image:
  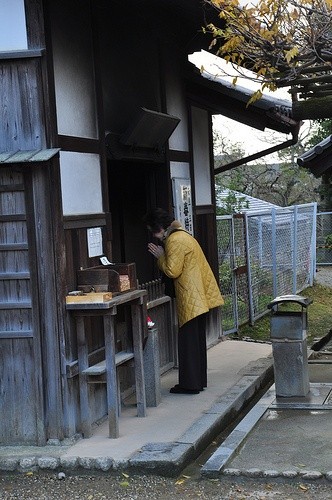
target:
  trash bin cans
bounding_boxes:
[267,292,314,398]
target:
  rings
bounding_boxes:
[153,250,154,253]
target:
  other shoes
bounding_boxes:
[170,384,203,394]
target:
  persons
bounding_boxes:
[148,208,224,394]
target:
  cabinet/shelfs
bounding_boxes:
[63,287,147,439]
